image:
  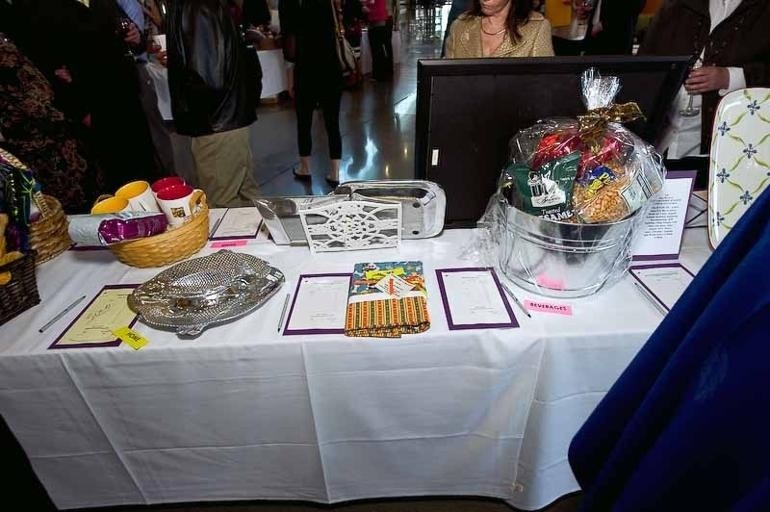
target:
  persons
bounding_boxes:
[0,1,769,212]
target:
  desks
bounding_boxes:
[551,24,587,56]
[144,48,288,122]
[0,207,714,511]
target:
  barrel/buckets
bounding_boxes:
[493,202,650,299]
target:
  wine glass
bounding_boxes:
[108,13,136,56]
[679,64,701,117]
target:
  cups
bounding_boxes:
[90,176,207,230]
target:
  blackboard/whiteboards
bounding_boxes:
[416,55,696,229]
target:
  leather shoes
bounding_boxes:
[292,167,311,182]
[325,175,339,187]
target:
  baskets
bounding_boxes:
[93,191,209,267]
[0,149,73,325]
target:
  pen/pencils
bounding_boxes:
[278,293,290,333]
[499,282,532,319]
[38,295,86,333]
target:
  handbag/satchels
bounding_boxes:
[335,34,361,86]
